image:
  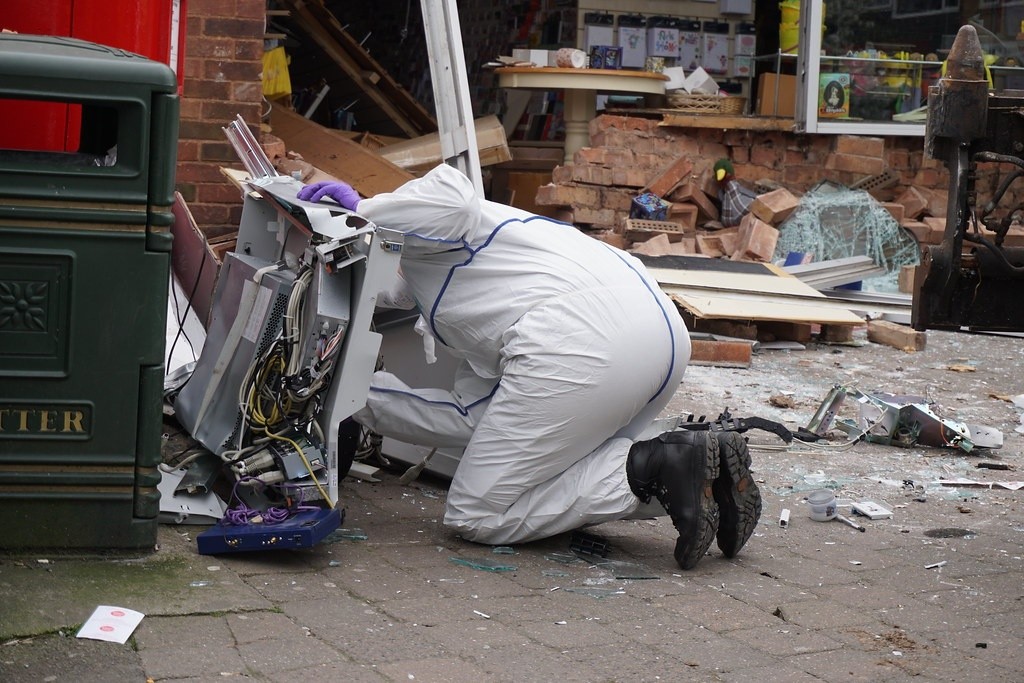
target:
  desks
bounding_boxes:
[745,51,1024,118]
[493,66,671,221]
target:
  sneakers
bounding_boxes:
[626,429,722,571]
[709,432,762,558]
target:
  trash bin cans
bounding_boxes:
[0,32,182,558]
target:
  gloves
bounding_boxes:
[296,181,361,213]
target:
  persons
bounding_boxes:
[294,163,762,569]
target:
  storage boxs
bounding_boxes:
[818,72,851,119]
[378,113,513,177]
[628,193,674,221]
[584,24,757,79]
[663,66,687,90]
[755,71,796,118]
[683,64,721,94]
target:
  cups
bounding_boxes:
[651,57,664,73]
[645,57,653,72]
[557,48,586,68]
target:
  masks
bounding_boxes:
[387,274,417,311]
